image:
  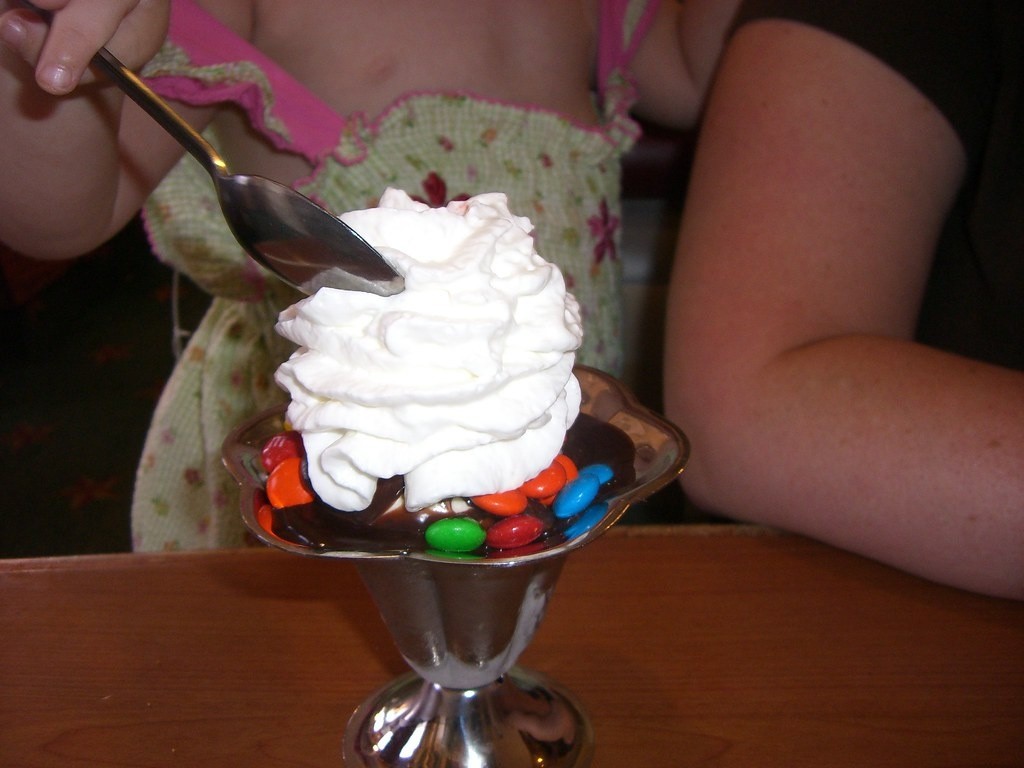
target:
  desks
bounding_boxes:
[0,524,1024,768]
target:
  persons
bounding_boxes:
[664,0,1024,603]
[0,0,743,553]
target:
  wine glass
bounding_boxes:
[217,375,690,768]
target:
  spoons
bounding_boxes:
[23,2,405,297]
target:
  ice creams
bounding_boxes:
[252,185,641,552]
